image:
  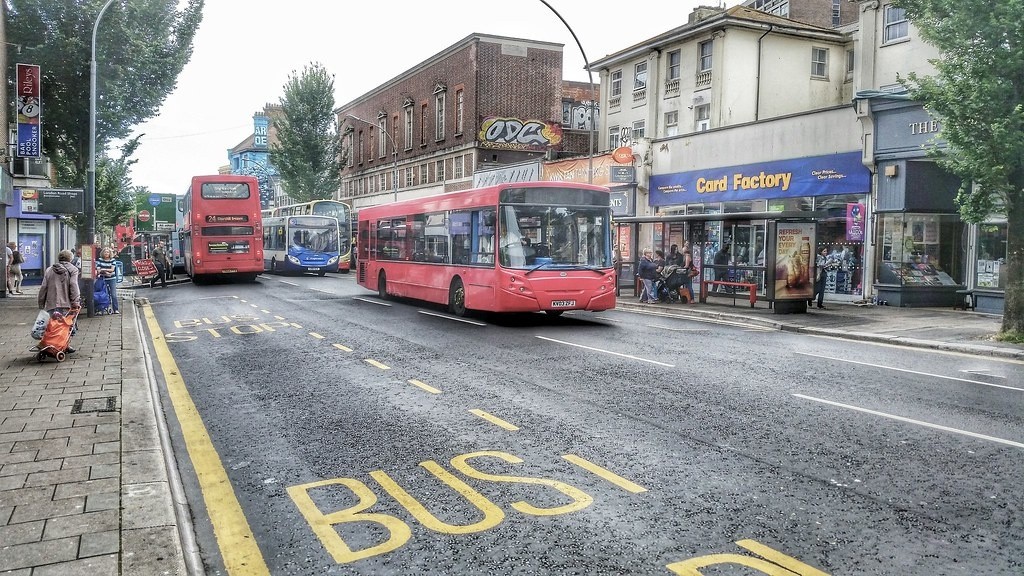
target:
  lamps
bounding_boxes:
[599,68,609,76]
[717,28,725,38]
[650,50,661,58]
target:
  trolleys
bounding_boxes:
[29,305,82,362]
[93,274,113,316]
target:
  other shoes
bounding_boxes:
[16,290,24,294]
[64,346,76,353]
[115,310,120,314]
[95,311,103,316]
[9,290,14,295]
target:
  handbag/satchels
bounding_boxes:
[31,310,50,339]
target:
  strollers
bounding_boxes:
[657,267,690,305]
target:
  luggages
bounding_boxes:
[30,306,81,362]
[94,274,113,315]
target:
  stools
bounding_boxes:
[953,290,975,311]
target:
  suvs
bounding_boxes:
[114,245,148,276]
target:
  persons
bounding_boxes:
[71,247,120,314]
[639,244,695,304]
[491,223,528,264]
[712,244,733,294]
[6,242,23,298]
[808,246,833,310]
[463,234,489,263]
[37,250,80,354]
[580,244,623,295]
[151,240,171,288]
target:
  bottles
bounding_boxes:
[800,236,811,283]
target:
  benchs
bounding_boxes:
[702,280,756,307]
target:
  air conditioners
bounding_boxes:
[0,148,11,164]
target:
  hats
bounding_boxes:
[7,242,16,247]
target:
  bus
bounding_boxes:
[172,173,265,286]
[134,231,172,253]
[262,215,341,276]
[356,180,617,319]
[261,199,352,275]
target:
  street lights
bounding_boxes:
[346,112,398,202]
[241,158,277,208]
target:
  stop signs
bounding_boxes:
[138,210,150,222]
